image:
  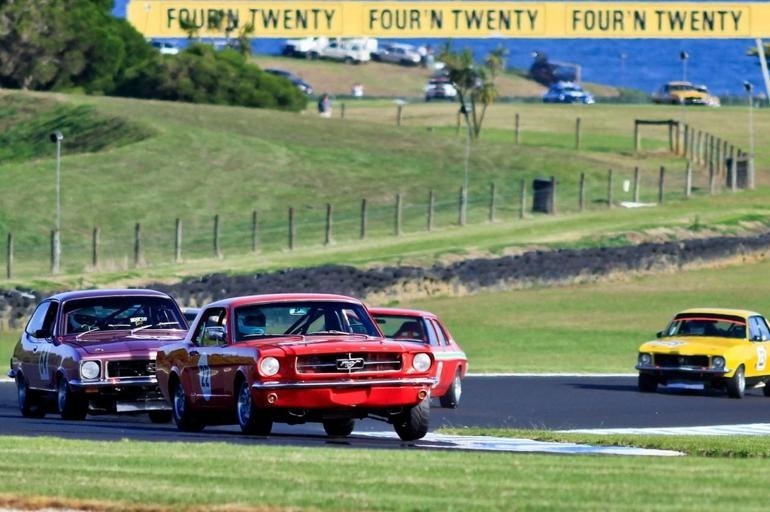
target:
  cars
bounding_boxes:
[635,307,770,398]
[424,77,457,101]
[652,81,708,105]
[7,288,191,423]
[320,308,470,409]
[696,86,722,107]
[265,70,314,98]
[541,83,594,104]
[370,43,422,67]
[177,307,264,338]
[154,293,438,441]
[530,64,582,81]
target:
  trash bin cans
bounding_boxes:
[726,158,747,189]
[533,178,552,213]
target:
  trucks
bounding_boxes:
[285,38,378,61]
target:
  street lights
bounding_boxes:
[619,53,627,98]
[744,80,754,188]
[680,51,689,81]
[49,129,64,275]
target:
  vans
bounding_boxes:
[152,42,179,55]
[319,42,370,65]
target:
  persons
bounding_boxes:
[317,92,335,120]
